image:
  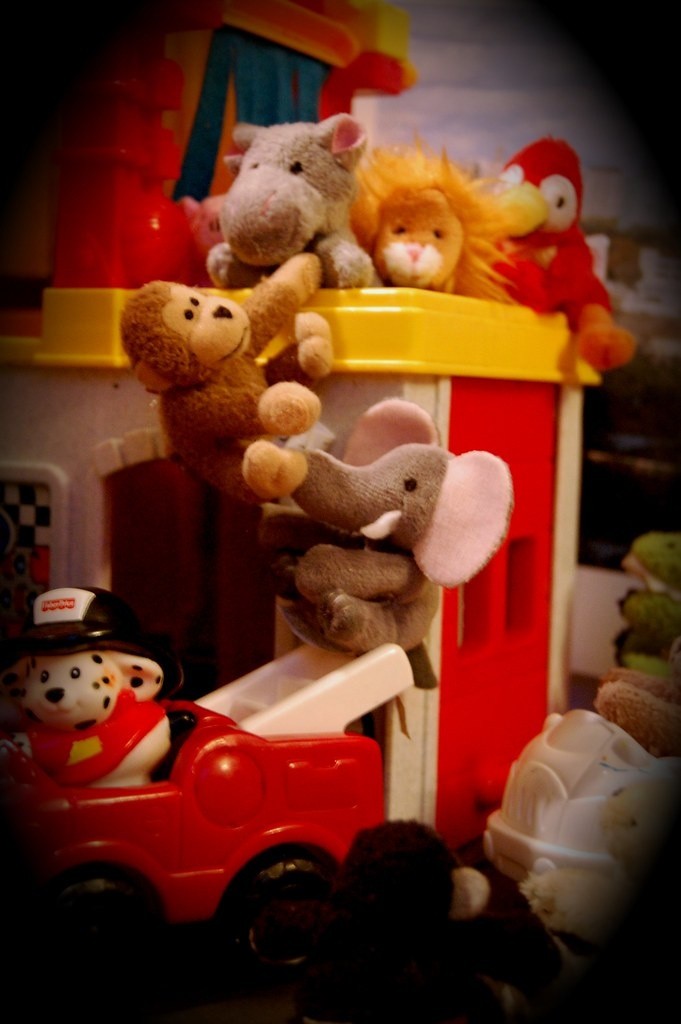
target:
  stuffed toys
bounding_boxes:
[518,757,681,946]
[207,112,382,287]
[353,145,511,302]
[0,587,186,784]
[183,194,225,247]
[249,399,513,687]
[296,821,490,1024]
[118,254,333,504]
[597,531,680,754]
[497,136,635,370]
